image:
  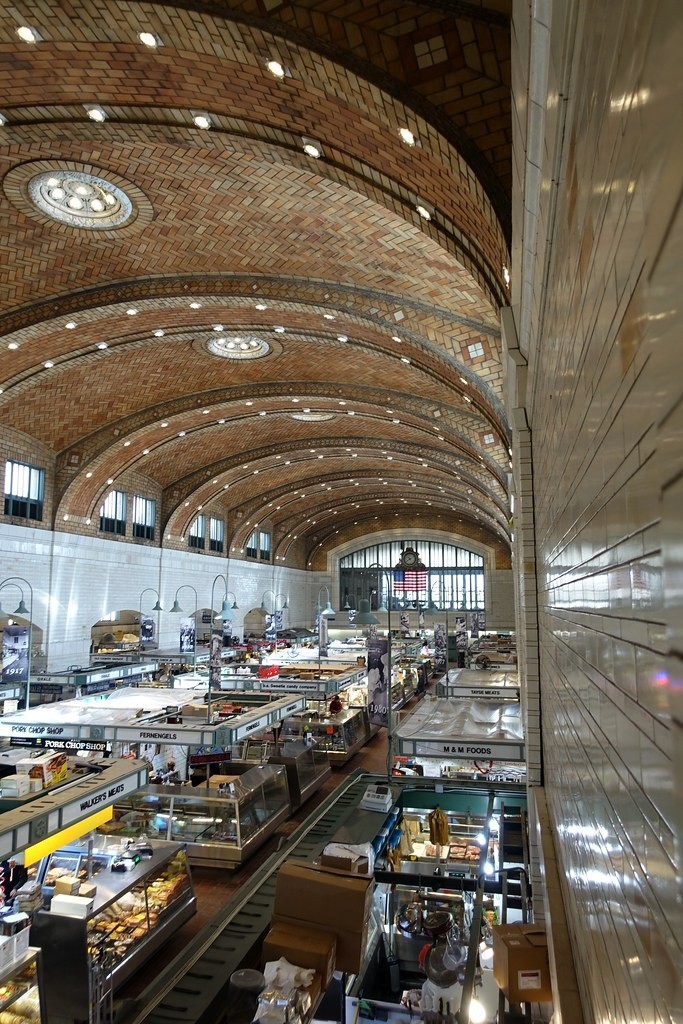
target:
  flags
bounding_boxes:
[393,570,426,592]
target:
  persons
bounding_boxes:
[0,859,27,910]
[422,638,429,652]
[285,643,292,648]
[391,665,393,676]
[152,666,206,682]
[329,695,343,715]
[417,670,424,702]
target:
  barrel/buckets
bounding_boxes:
[227,969,265,1024]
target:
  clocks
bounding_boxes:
[402,551,417,566]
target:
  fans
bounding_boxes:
[473,654,491,671]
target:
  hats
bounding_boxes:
[335,695,339,700]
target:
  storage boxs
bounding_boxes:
[50,875,98,916]
[181,704,212,716]
[496,638,510,644]
[256,840,374,1024]
[299,672,314,680]
[492,923,553,1003]
[0,748,67,798]
[119,826,141,837]
